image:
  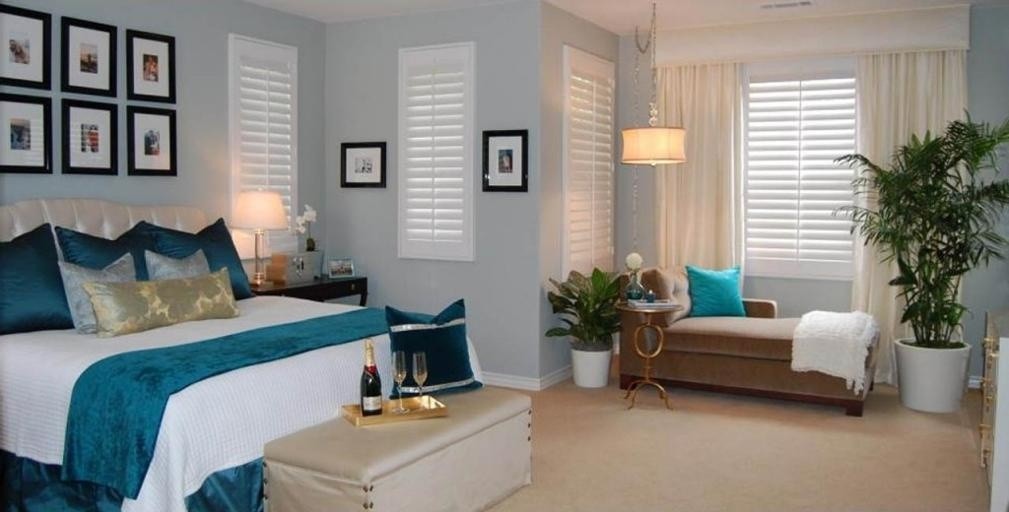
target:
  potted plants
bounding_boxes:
[826,101,1009,416]
[543,265,629,389]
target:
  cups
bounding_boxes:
[646,294,656,302]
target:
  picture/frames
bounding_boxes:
[339,139,389,190]
[480,127,531,195]
[60,98,121,178]
[324,254,359,281]
[58,4,122,97]
[126,103,178,178]
[0,91,58,177]
[126,7,185,106]
[1,1,54,92]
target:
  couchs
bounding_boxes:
[621,263,885,418]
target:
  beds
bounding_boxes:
[2,193,494,512]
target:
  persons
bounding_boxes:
[7,35,162,156]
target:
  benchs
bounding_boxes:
[260,384,538,511]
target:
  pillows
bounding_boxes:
[639,263,694,327]
[0,221,263,337]
[381,295,487,400]
[684,264,750,317]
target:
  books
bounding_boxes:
[627,298,680,311]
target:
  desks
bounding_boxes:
[614,297,685,414]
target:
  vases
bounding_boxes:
[302,251,324,279]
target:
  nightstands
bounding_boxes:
[250,277,374,308]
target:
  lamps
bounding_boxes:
[619,3,697,169]
[227,184,289,286]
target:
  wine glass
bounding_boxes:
[409,352,433,411]
[389,351,412,414]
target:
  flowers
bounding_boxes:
[293,201,323,253]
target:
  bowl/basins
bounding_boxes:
[627,290,643,299]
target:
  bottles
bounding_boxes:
[359,338,385,418]
